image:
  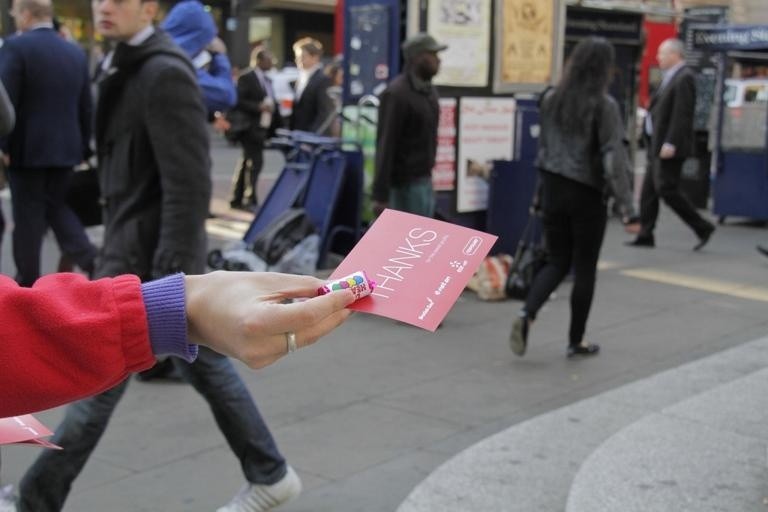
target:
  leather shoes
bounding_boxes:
[626,237,656,248]
[694,223,715,251]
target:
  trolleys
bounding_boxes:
[211,94,381,281]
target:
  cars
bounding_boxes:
[226,64,299,135]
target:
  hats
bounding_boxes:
[404,35,448,55]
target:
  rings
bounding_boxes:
[287,332,298,355]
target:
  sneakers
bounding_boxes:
[568,342,600,357]
[217,465,302,512]
[510,312,528,355]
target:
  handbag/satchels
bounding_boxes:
[69,165,103,228]
[505,240,552,302]
[225,107,251,142]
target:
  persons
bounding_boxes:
[160,1,236,109]
[1,0,101,287]
[370,31,448,217]
[0,0,302,512]
[224,47,284,212]
[209,110,229,133]
[623,38,716,251]
[509,35,641,360]
[0,270,356,418]
[287,38,345,134]
